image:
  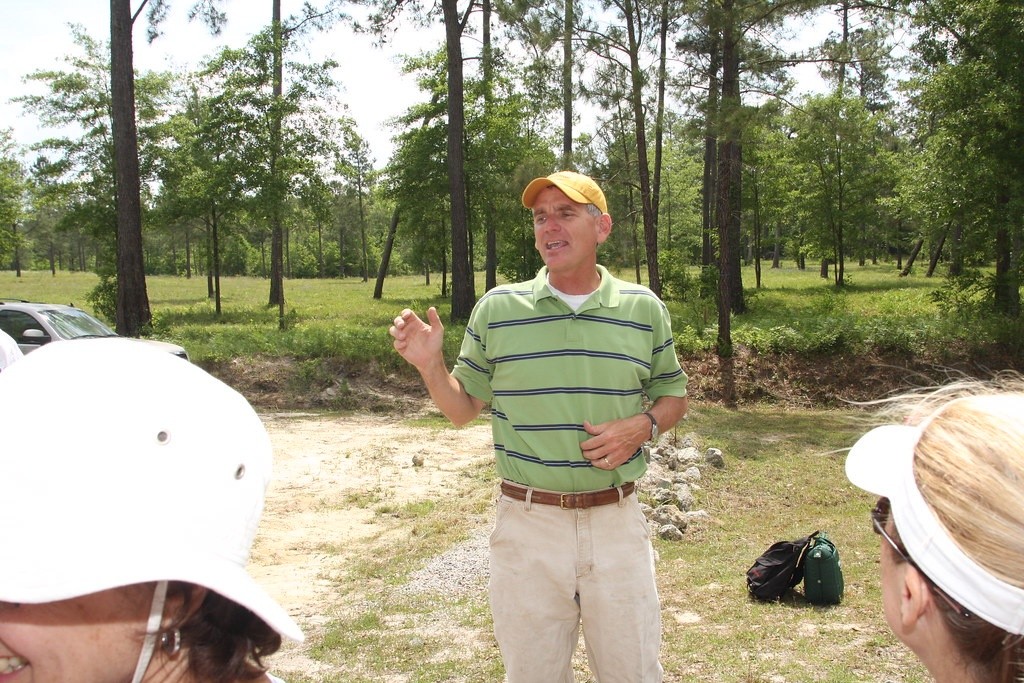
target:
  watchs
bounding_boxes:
[645,411,658,442]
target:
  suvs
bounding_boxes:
[0,297,190,363]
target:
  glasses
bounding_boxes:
[872,494,967,621]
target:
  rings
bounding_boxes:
[604,458,612,468]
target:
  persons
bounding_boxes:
[845,372,1024,683]
[0,336,304,683]
[388,172,690,683]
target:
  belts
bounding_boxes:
[497,482,638,509]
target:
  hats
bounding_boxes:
[844,402,1024,632]
[522,170,606,213]
[0,340,303,638]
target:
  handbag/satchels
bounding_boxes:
[747,529,844,607]
